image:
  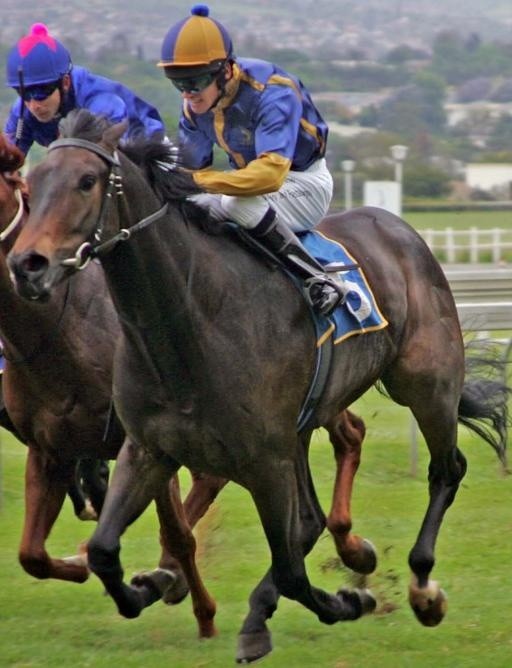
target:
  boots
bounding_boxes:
[253,211,349,320]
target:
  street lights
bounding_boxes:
[340,158,356,212]
[388,144,409,220]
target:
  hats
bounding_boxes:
[6,22,74,87]
[156,3,233,79]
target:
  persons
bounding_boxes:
[156,4,347,317]
[3,22,166,165]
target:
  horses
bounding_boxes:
[8,111,512,663]
[1,135,380,642]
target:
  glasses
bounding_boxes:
[167,39,232,95]
[14,81,60,105]
